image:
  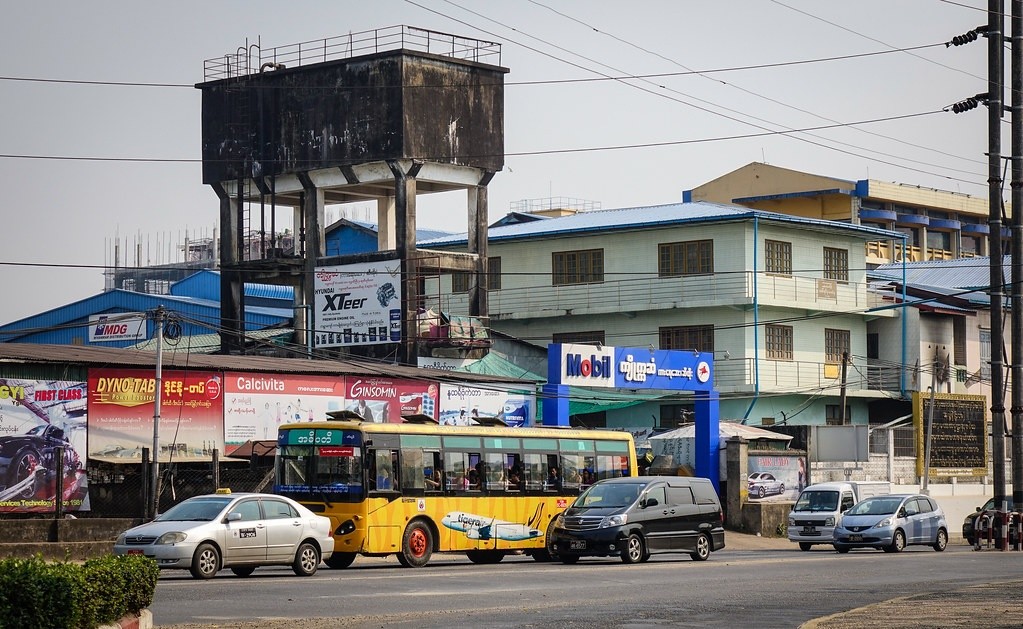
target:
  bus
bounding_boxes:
[251,410,639,569]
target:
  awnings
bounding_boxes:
[450,352,673,420]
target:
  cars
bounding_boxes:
[963,496,1013,545]
[833,494,949,553]
[748,471,786,498]
[114,488,335,579]
[0,425,80,502]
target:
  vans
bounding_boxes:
[555,476,725,564]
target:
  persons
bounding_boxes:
[444,408,478,426]
[377,463,623,491]
[798,460,806,496]
[263,398,313,439]
[354,399,374,422]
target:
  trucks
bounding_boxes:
[787,481,891,551]
